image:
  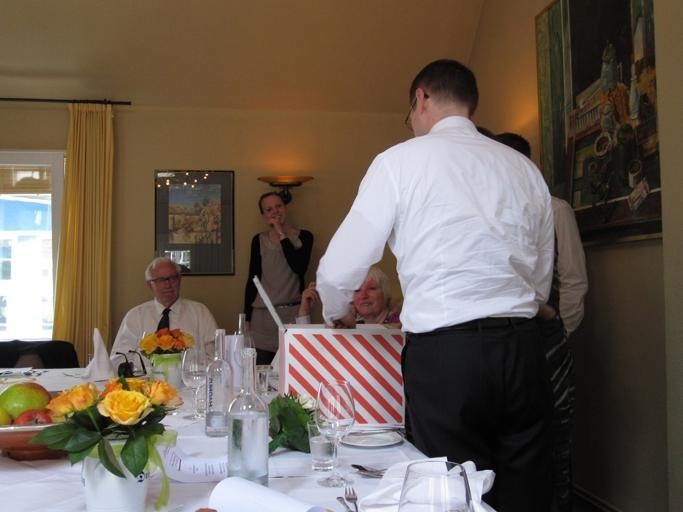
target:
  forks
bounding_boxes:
[344,487,358,511]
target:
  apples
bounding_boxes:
[0,410,12,425]
[1,381,52,422]
[14,409,51,427]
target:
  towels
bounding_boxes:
[84,328,115,382]
[360,457,496,507]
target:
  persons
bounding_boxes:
[296,267,391,325]
[496,129,590,511]
[316,58,553,512]
[244,192,315,366]
[110,257,220,379]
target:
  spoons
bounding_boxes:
[350,464,385,476]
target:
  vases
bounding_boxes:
[82,439,170,512]
[150,353,182,386]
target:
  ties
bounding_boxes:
[157,308,171,332]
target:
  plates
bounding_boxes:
[339,431,402,447]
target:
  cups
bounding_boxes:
[255,365,268,393]
[306,420,337,471]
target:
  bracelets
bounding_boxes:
[277,232,285,237]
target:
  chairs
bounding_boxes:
[0,340,81,368]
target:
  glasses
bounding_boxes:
[405,93,429,135]
[148,275,180,283]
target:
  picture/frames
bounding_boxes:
[155,168,236,276]
[536,4,670,243]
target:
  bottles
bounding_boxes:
[205,328,232,441]
[236,312,251,352]
[224,348,270,489]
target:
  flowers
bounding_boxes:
[28,372,178,479]
[136,327,194,356]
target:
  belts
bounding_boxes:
[406,317,529,335]
[275,301,301,308]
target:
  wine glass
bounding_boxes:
[181,350,205,420]
[397,461,475,511]
[233,329,255,389]
[315,381,354,487]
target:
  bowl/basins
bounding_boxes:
[0,423,66,461]
